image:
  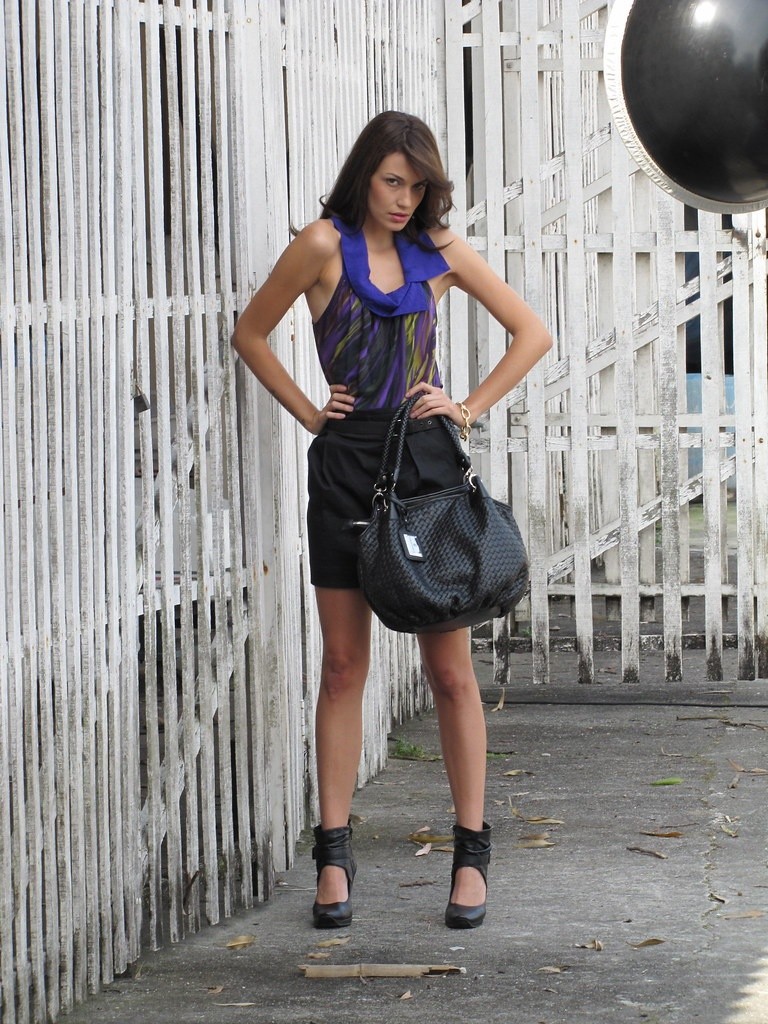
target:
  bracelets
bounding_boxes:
[454,401,472,441]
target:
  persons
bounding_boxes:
[230,111,554,928]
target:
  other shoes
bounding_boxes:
[313,823,357,929]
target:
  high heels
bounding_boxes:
[446,823,492,931]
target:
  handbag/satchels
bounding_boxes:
[361,391,529,633]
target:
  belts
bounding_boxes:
[329,417,440,436]
